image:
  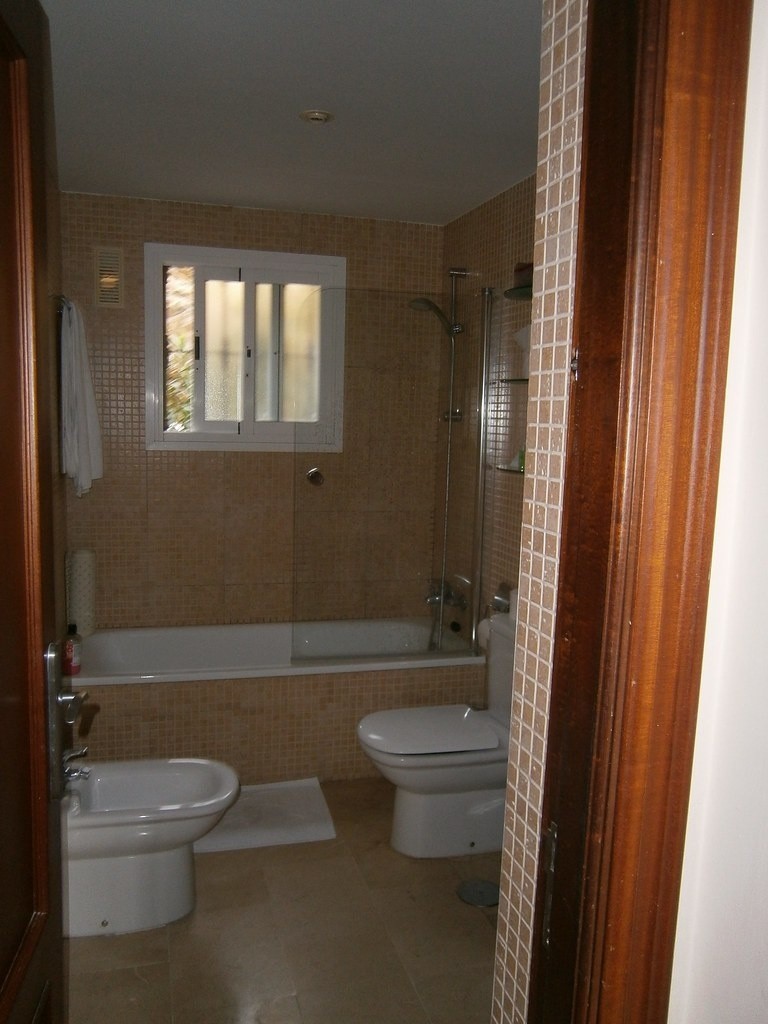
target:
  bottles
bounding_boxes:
[60,623,83,676]
[518,440,526,473]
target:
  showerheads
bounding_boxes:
[407,295,457,340]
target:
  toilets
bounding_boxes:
[355,618,518,860]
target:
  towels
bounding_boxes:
[56,300,105,500]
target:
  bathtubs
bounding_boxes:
[56,616,489,687]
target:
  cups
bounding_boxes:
[515,263,533,286]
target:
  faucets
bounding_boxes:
[58,742,92,789]
[424,578,457,609]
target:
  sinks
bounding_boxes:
[67,758,242,856]
